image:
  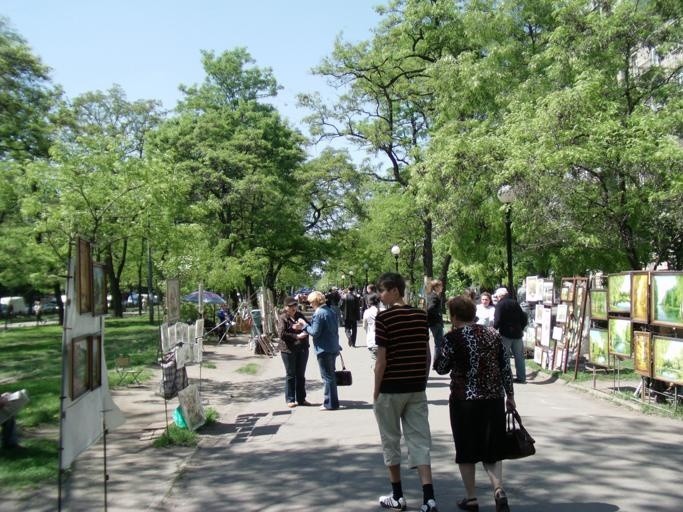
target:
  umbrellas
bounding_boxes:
[179,289,225,305]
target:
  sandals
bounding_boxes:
[457,498,479,512]
[494,487,510,512]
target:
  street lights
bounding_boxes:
[320,264,374,295]
[494,182,520,307]
[389,244,401,277]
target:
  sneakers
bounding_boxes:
[419,498,437,512]
[379,496,406,511]
[298,400,311,405]
[287,402,296,407]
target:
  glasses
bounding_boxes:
[378,288,387,294]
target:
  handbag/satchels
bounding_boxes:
[334,352,352,385]
[503,407,535,459]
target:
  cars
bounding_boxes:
[0,283,160,325]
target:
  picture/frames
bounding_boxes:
[608,316,633,359]
[650,271,683,329]
[89,331,101,391]
[589,328,609,367]
[630,271,649,324]
[72,334,89,402]
[78,237,91,315]
[608,273,630,313]
[91,262,108,316]
[653,335,683,386]
[633,331,652,377]
[525,276,583,370]
[589,289,608,320]
[167,279,180,321]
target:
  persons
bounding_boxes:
[291,291,342,411]
[215,304,226,343]
[372,272,441,512]
[31,300,45,327]
[430,296,517,511]
[275,295,312,409]
[319,277,527,385]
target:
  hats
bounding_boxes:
[283,295,298,307]
